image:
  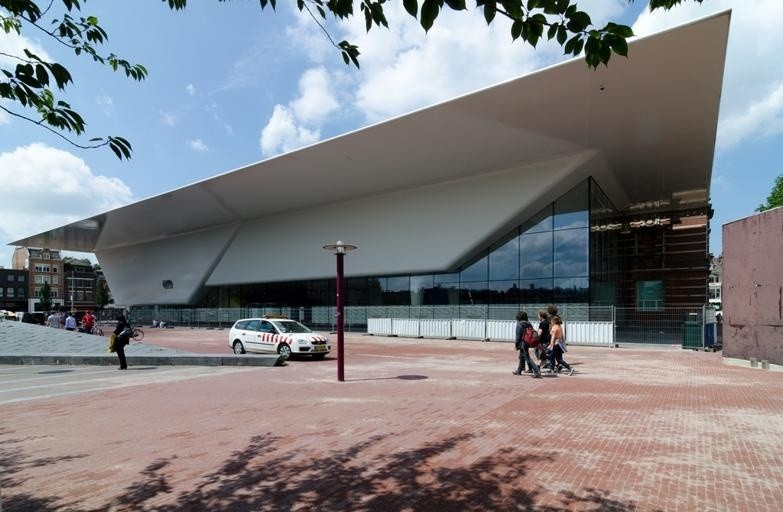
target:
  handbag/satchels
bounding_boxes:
[117,327,132,343]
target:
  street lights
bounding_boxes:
[321,240,358,381]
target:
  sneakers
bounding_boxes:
[512,362,574,378]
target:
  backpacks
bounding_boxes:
[522,328,538,347]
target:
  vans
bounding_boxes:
[20,313,45,325]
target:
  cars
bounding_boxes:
[228,315,332,360]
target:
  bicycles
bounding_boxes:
[130,327,144,341]
[90,327,103,336]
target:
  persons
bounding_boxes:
[59,312,64,328]
[81,310,94,334]
[538,306,574,376]
[46,310,58,328]
[113,315,129,370]
[64,312,76,331]
[511,311,541,378]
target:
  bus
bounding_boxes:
[0,310,17,321]
